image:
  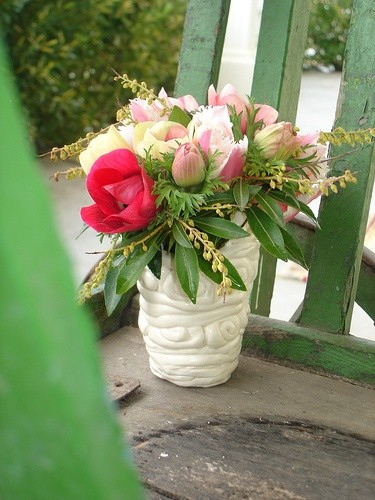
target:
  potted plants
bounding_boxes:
[30,65,375,318]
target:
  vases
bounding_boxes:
[134,212,263,388]
